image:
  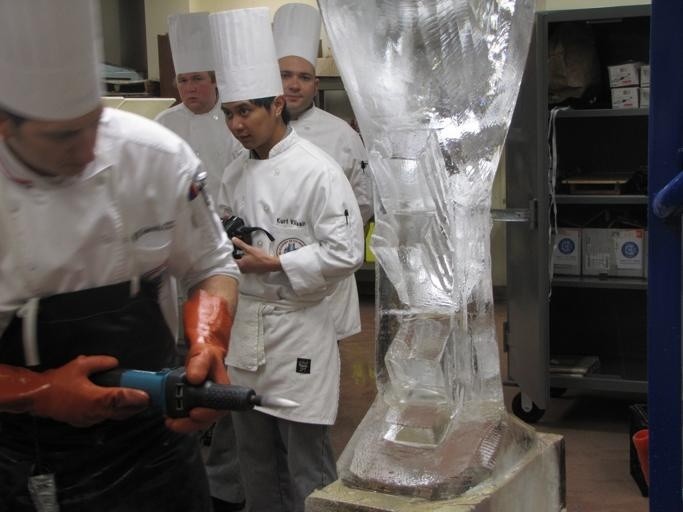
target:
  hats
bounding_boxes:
[165,2,321,103]
[0,1,103,123]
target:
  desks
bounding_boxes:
[294,425,567,512]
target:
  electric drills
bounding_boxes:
[87,367,301,420]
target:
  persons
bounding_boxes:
[153,11,252,204]
[0,1,241,512]
[206,6,364,512]
[271,3,370,339]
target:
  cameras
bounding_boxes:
[222,216,252,259]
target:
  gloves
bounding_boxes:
[0,356,151,427]
[166,290,232,438]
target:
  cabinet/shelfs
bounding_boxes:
[527,2,653,396]
[313,74,381,282]
